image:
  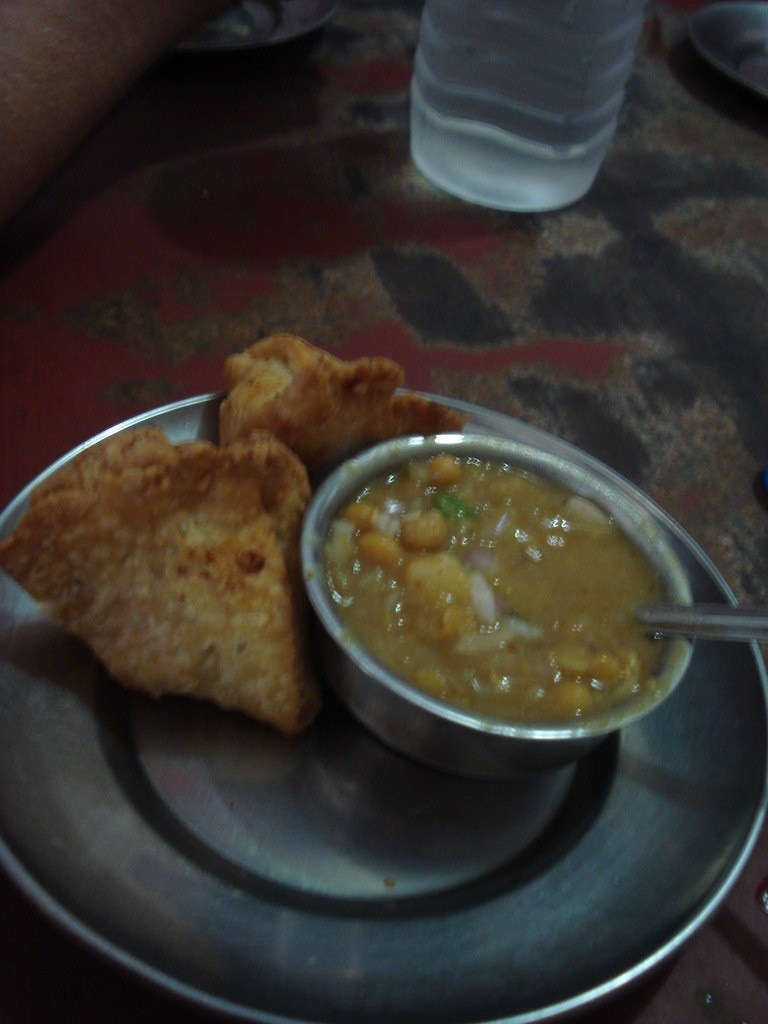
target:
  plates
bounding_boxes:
[691,1,768,103]
[3,379,768,1024]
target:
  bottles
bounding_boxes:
[404,1,650,215]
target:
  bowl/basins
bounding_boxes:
[298,435,704,784]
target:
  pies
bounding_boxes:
[215,334,467,492]
[0,426,327,730]
